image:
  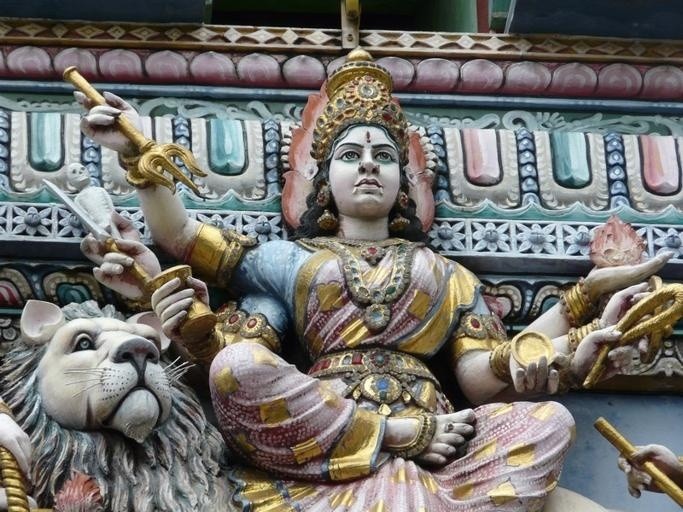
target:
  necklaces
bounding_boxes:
[301,237,424,333]
[331,234,409,262]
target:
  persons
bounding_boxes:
[71,45,673,511]
[0,394,40,511]
[617,440,682,500]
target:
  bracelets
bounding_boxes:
[557,276,596,328]
[182,331,228,364]
[134,297,153,313]
[118,146,164,189]
[489,341,519,385]
[567,318,600,350]
